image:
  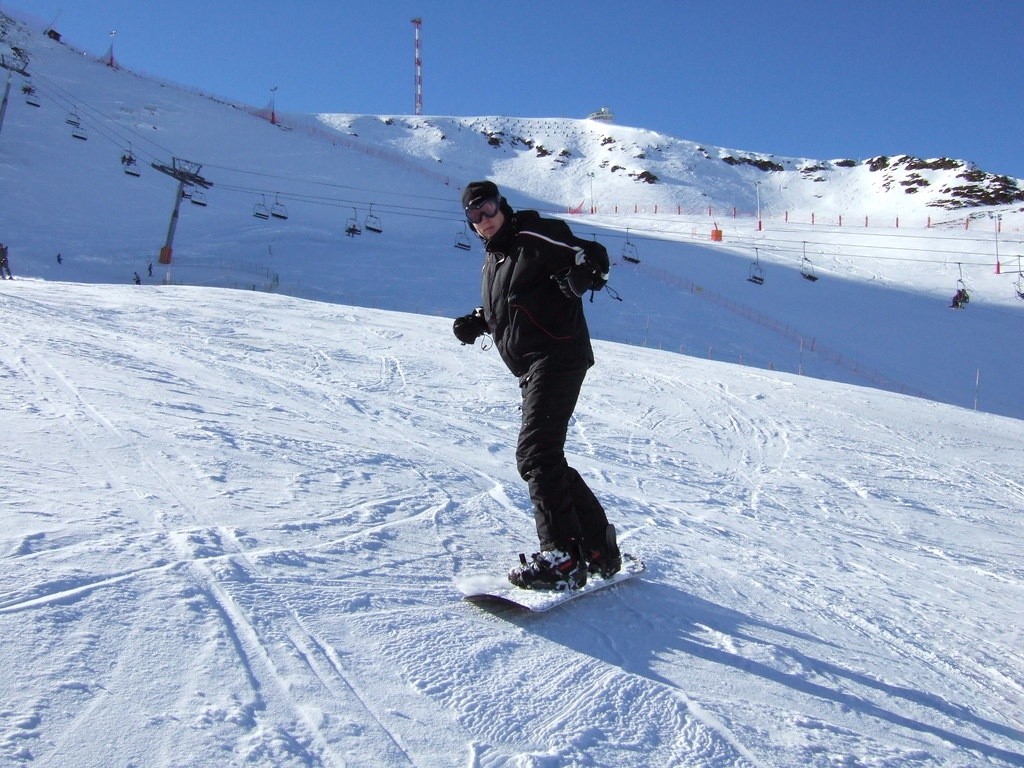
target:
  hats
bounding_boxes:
[462,180,498,213]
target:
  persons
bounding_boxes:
[345,224,356,238]
[57,252,63,265]
[949,289,969,308]
[22,86,32,95]
[148,262,154,278]
[0,241,16,281]
[120,154,134,166]
[132,271,142,285]
[452,178,639,593]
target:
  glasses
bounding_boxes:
[465,198,501,223]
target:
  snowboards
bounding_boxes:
[465,550,644,613]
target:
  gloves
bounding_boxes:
[454,314,484,345]
[550,266,595,299]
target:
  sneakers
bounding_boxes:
[580,524,622,580]
[509,549,585,590]
